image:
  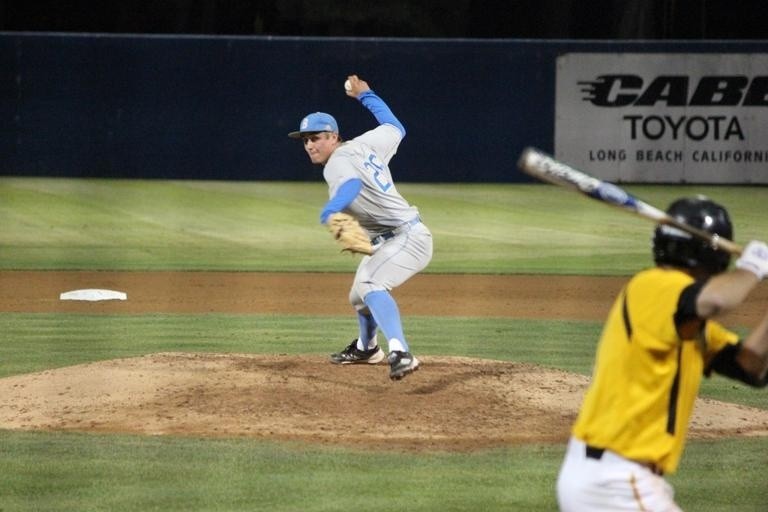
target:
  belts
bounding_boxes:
[371,217,421,245]
[586,446,604,459]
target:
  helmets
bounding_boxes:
[653,195,732,276]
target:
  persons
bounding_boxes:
[553,193,767,512]
[286,69,433,379]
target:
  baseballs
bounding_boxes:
[345,80,351,91]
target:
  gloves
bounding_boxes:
[736,240,768,281]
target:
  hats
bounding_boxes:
[288,112,339,138]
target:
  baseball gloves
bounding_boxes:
[325,213,374,256]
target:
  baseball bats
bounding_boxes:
[519,147,745,254]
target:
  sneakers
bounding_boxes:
[386,350,420,379]
[330,339,385,365]
